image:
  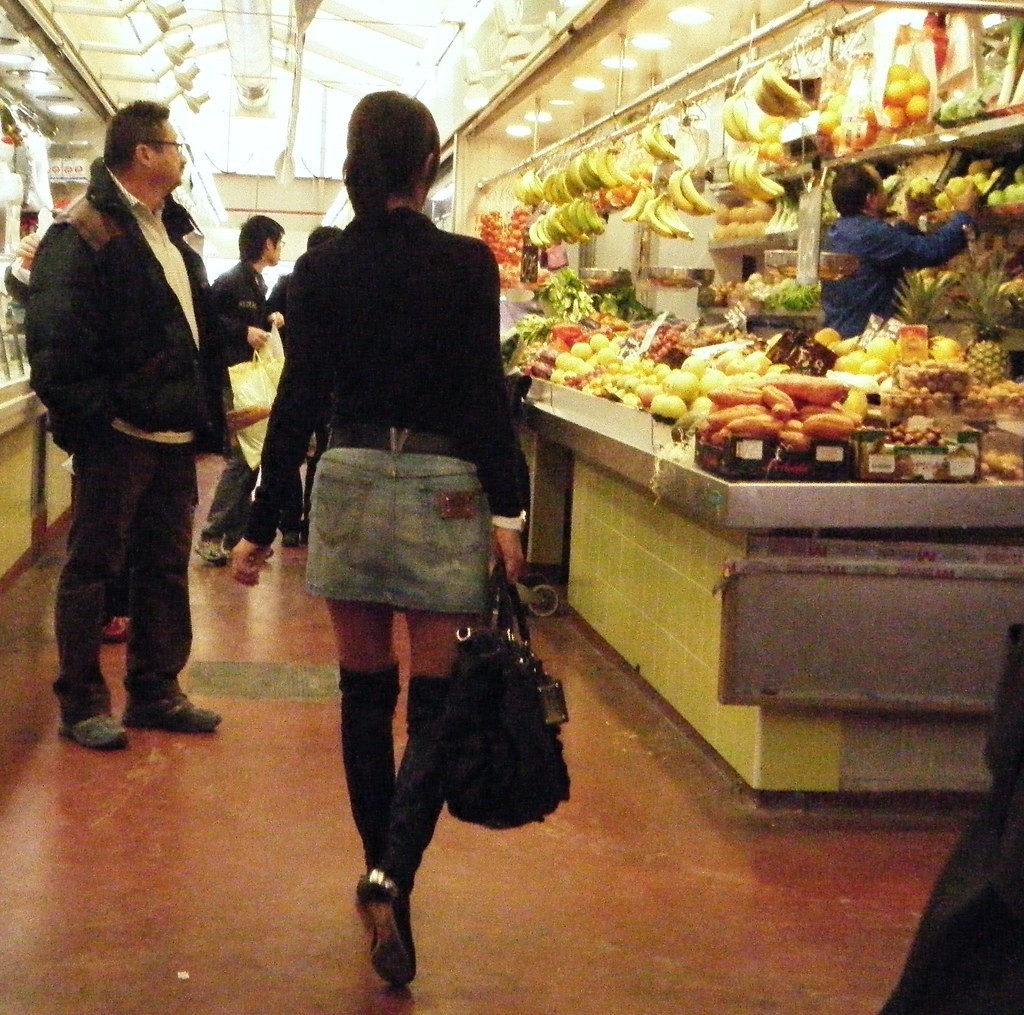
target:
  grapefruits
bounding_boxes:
[550,336,616,387]
[651,332,963,420]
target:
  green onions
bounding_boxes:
[997,17,1024,108]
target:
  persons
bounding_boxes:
[818,162,983,344]
[19,94,223,749]
[231,88,529,990]
[3,157,344,564]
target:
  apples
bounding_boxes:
[906,157,1024,209]
[590,359,671,408]
[476,207,540,289]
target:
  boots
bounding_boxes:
[358,677,458,986]
[338,665,402,871]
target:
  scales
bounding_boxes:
[763,66,861,287]
[632,118,715,291]
[577,212,631,289]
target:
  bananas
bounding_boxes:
[515,59,811,239]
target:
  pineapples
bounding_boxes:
[959,258,1022,384]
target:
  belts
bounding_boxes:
[327,424,476,463]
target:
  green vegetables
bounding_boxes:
[516,269,663,338]
[764,283,824,314]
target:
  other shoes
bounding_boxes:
[283,531,299,546]
[102,616,130,639]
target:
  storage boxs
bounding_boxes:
[852,431,982,484]
[688,425,861,476]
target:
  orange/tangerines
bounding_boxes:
[817,64,932,147]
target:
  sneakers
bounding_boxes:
[194,539,228,561]
[123,692,222,731]
[59,715,129,749]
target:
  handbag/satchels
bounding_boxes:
[431,563,570,830]
[259,324,286,386]
[227,349,280,469]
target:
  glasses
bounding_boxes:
[137,137,185,158]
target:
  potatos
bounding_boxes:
[697,374,856,454]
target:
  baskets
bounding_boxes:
[694,428,848,481]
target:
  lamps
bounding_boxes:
[50,0,210,113]
